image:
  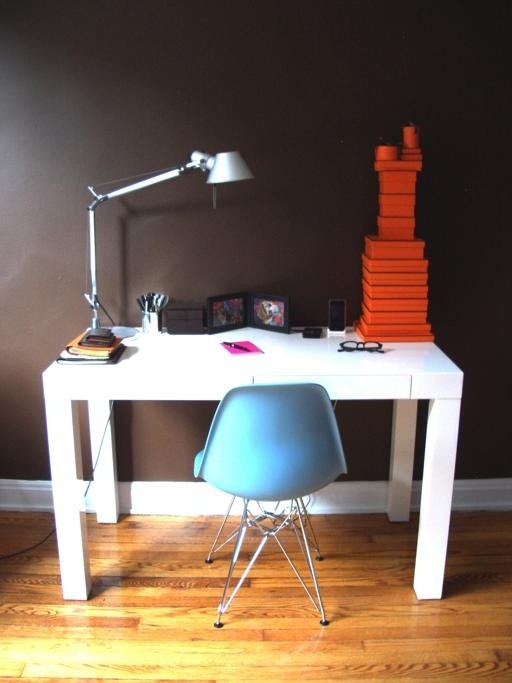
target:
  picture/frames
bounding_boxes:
[208,292,248,332]
[249,295,292,333]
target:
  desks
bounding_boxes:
[41,322,469,600]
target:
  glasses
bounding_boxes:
[338,340,384,354]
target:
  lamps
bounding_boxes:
[76,146,256,346]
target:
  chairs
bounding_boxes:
[192,383,345,626]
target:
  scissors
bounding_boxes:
[153,292,169,312]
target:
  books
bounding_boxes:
[56,327,127,367]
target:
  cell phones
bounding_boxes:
[328,300,346,331]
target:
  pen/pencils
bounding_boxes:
[136,292,155,313]
[224,341,248,351]
